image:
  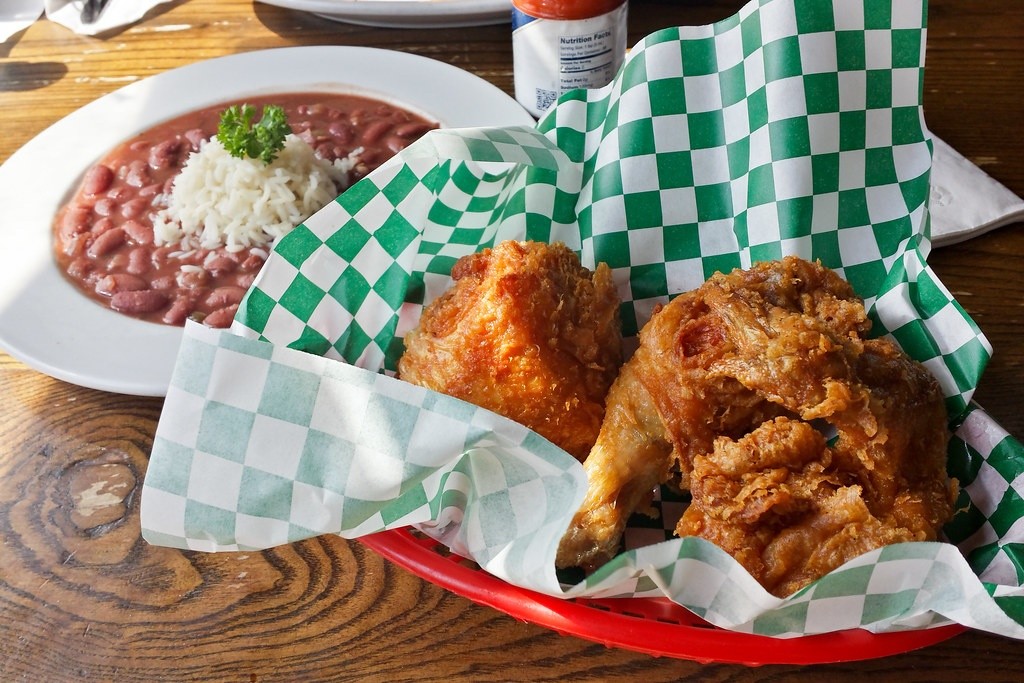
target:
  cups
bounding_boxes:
[511,0,627,120]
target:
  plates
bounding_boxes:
[0,45,538,399]
[361,407,1024,665]
[255,1,512,23]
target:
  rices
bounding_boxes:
[151,124,359,261]
